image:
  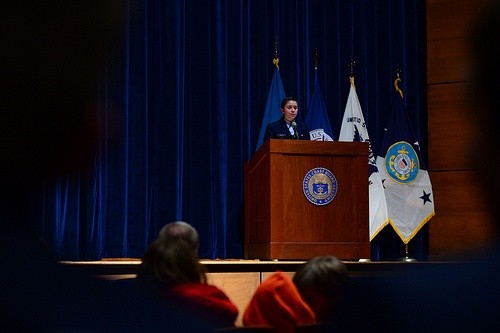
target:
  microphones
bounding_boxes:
[291,122,299,139]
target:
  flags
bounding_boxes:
[341,76,390,242]
[387,79,435,244]
[255,66,304,153]
[301,68,337,141]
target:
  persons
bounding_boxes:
[263,97,311,144]
[128,220,497,331]
